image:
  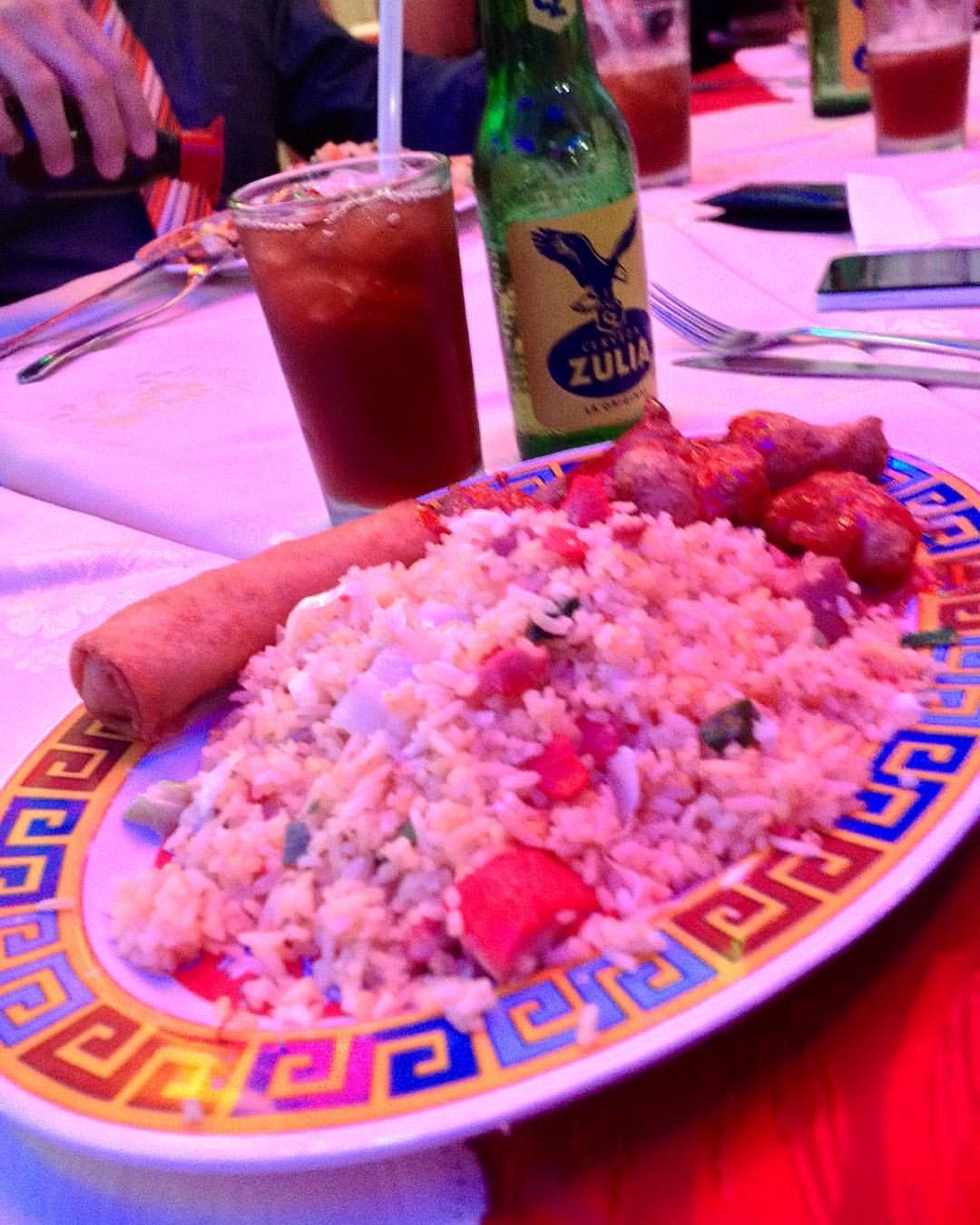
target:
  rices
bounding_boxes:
[97,492,948,1030]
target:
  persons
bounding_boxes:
[0,1,483,304]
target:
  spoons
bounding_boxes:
[18,251,232,381]
[1,239,200,360]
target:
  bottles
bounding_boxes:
[808,0,874,118]
[469,0,659,461]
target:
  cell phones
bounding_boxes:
[815,248,980,310]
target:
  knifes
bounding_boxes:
[674,351,980,392]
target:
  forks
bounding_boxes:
[648,274,979,366]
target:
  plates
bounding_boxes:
[1,431,978,1171]
[138,208,249,277]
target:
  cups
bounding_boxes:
[230,149,490,531]
[866,1,976,157]
[584,0,702,185]
